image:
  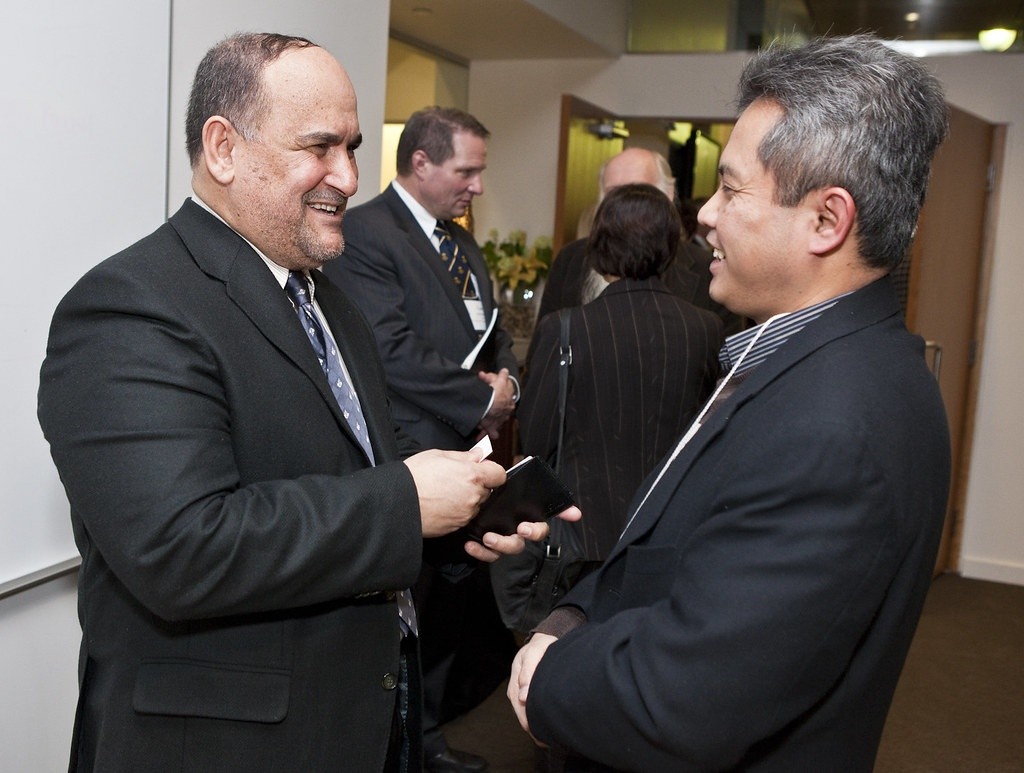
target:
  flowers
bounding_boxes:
[479,228,550,292]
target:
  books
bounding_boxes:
[422,456,575,575]
[461,308,501,371]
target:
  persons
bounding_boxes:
[322,105,522,722]
[37,32,582,773]
[506,36,951,773]
[521,147,743,579]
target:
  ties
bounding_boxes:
[433,220,483,342]
[285,269,419,638]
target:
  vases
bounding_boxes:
[497,303,533,339]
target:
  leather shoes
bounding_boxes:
[425,747,489,773]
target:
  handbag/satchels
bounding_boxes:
[487,518,587,636]
